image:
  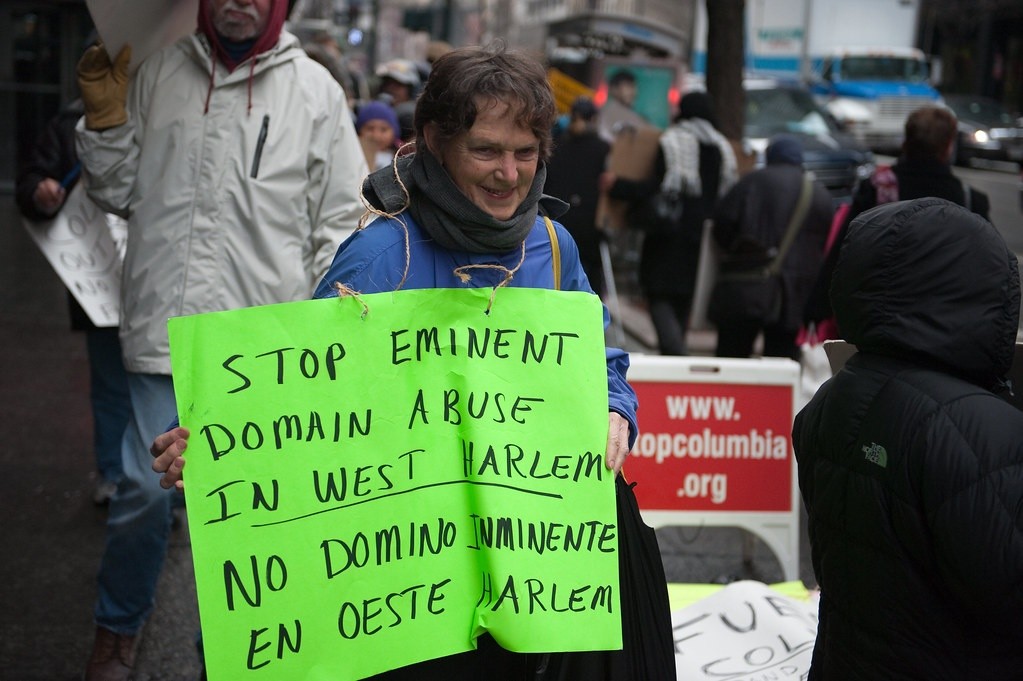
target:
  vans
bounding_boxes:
[810,45,949,151]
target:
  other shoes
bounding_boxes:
[90,474,118,505]
[84,626,143,681]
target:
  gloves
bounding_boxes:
[74,39,132,130]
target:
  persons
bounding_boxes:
[1,0,1022,681]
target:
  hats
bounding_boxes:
[355,101,400,136]
[375,58,421,85]
[679,92,711,119]
[765,140,804,165]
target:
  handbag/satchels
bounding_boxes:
[706,261,789,334]
[799,321,848,418]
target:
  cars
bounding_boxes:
[943,91,1023,168]
[743,75,881,213]
